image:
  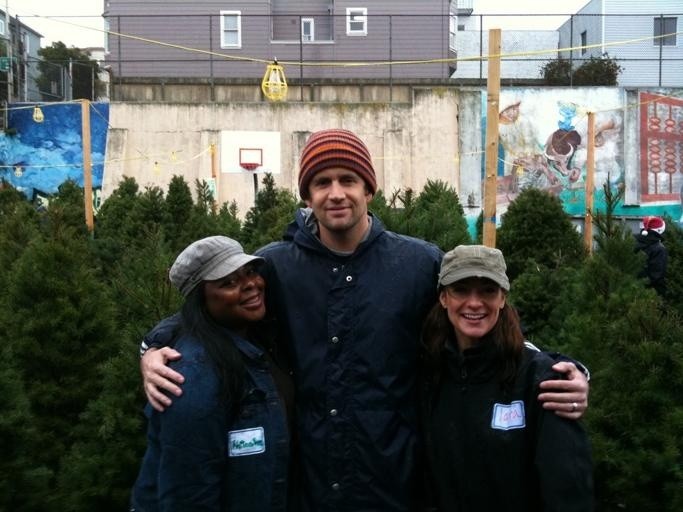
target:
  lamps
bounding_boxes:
[14,167,22,177]
[261,57,288,101]
[515,166,524,176]
[31,105,44,122]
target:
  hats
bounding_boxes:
[639,215,666,236]
[167,233,265,300]
[435,243,511,293]
[297,127,377,200]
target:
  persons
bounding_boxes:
[129,233,299,512]
[137,127,598,509]
[414,240,598,511]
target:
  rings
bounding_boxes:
[568,400,579,414]
